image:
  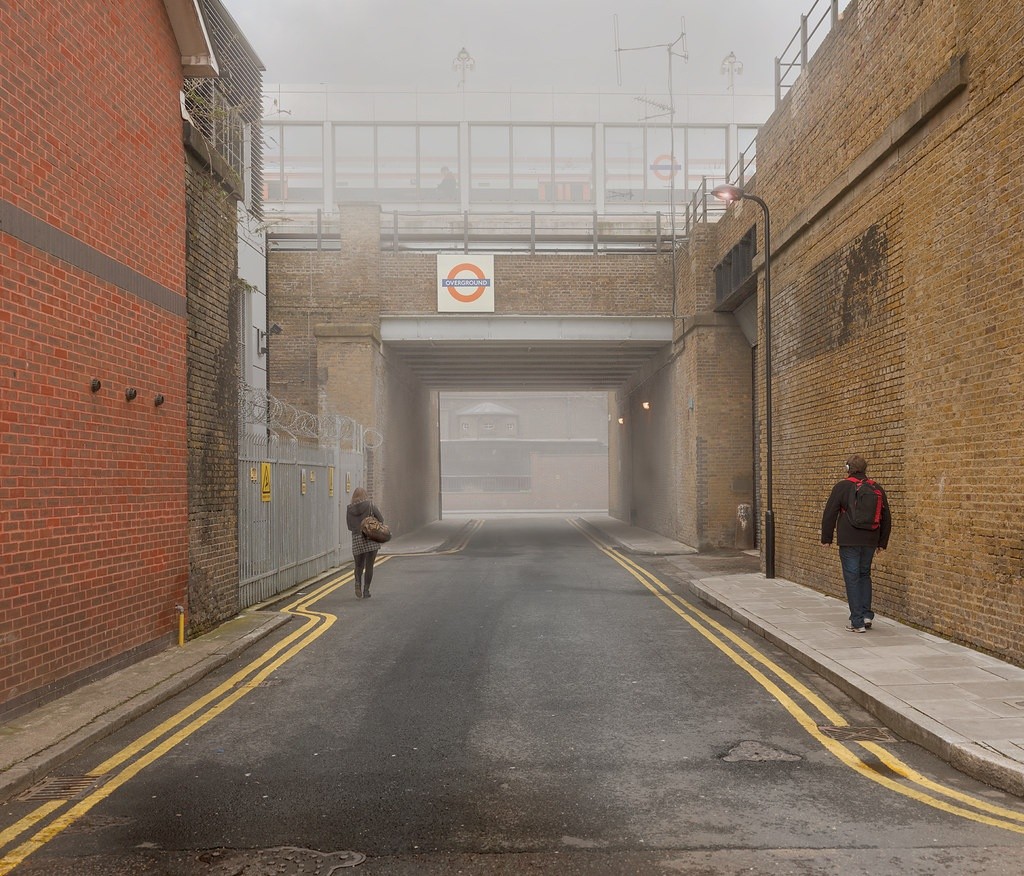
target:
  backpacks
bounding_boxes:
[842,477,883,530]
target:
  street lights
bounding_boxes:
[711,183,775,578]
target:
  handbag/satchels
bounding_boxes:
[361,501,392,543]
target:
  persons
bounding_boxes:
[820,454,892,633]
[346,487,384,597]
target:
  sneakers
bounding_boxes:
[845,624,866,633]
[864,618,872,626]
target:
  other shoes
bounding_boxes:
[355,582,362,597]
[364,590,372,598]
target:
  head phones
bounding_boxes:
[844,461,849,472]
